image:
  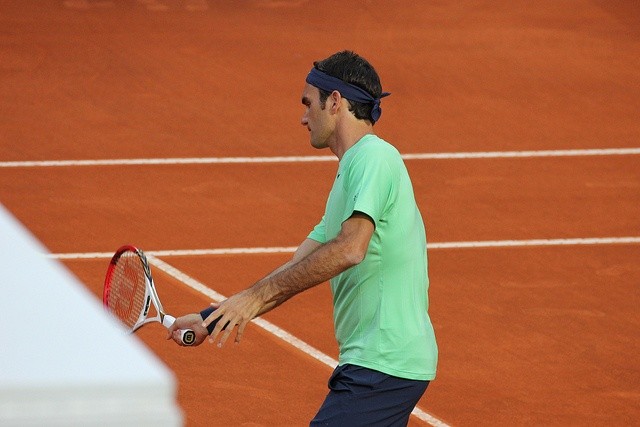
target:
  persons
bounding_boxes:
[165,49,439,426]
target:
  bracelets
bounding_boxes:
[199,303,227,336]
